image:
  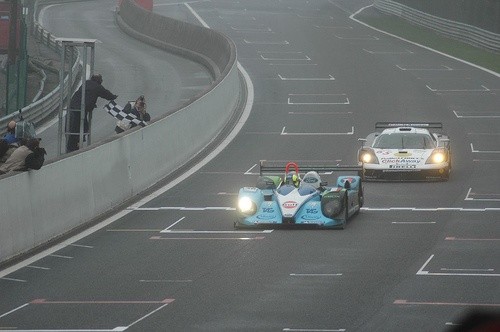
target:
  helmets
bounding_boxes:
[301,170,322,189]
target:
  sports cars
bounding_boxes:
[235,162,363,231]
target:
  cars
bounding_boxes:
[358,120,451,183]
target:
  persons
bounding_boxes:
[3,120,21,144]
[64,72,119,153]
